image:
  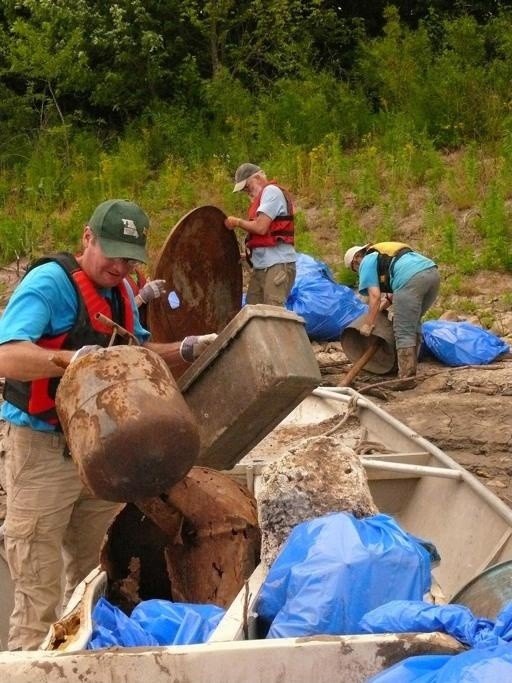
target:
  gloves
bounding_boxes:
[179,334,218,362]
[137,278,167,304]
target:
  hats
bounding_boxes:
[233,164,261,194]
[88,199,148,266]
[345,243,369,270]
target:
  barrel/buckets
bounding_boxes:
[341,307,397,377]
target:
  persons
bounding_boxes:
[124,260,168,329]
[0,199,219,652]
[344,242,441,390]
[225,164,295,307]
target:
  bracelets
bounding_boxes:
[237,218,241,227]
